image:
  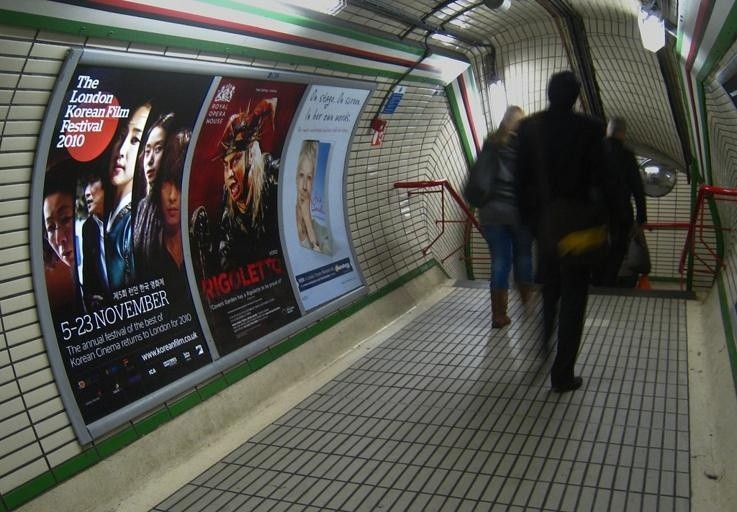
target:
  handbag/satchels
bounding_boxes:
[537,192,609,276]
[463,155,499,207]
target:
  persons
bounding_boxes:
[592,116,648,288]
[150,154,187,288]
[468,105,535,328]
[130,103,178,279]
[101,95,169,288]
[193,111,280,282]
[512,71,610,392]
[615,216,652,288]
[292,138,330,254]
[46,155,97,317]
[78,166,116,311]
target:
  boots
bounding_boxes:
[492,287,510,327]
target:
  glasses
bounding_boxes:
[44,216,73,240]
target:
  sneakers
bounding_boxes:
[551,377,582,393]
